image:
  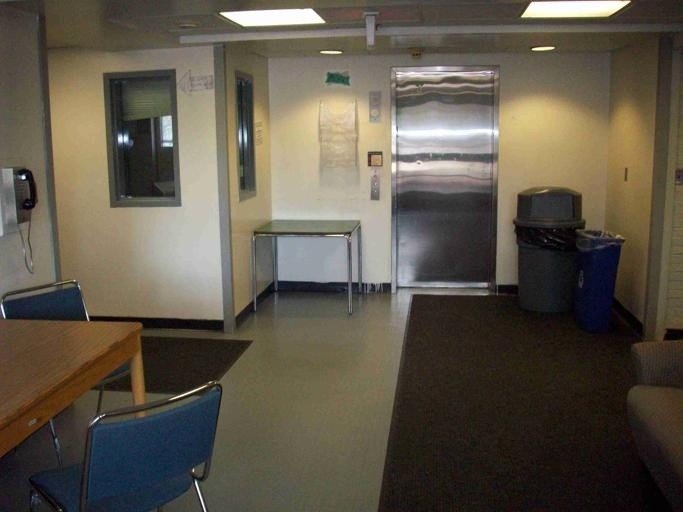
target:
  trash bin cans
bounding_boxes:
[513,186,625,334]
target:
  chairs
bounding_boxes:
[1,280,131,467]
[29,380,223,512]
[627,340,683,512]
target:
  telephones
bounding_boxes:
[1,165,37,226]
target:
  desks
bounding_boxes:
[1,319,146,458]
[252,219,362,315]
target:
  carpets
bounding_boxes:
[377,294,674,512]
[91,335,253,395]
